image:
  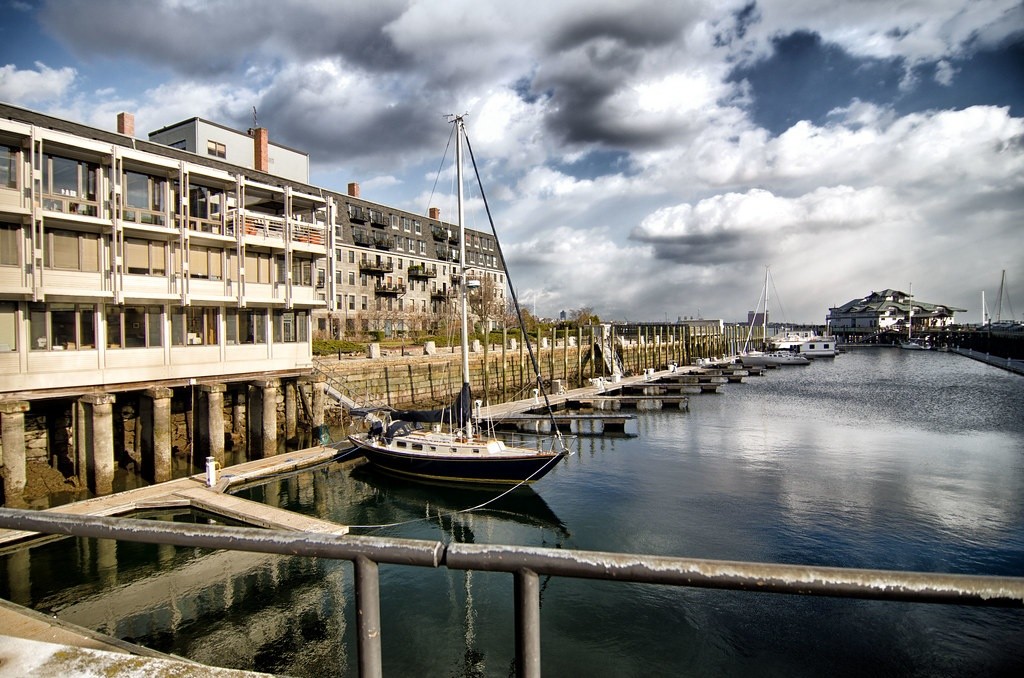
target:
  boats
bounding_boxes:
[348,112,573,493]
[741,266,847,367]
[899,282,932,349]
[977,270,1024,332]
